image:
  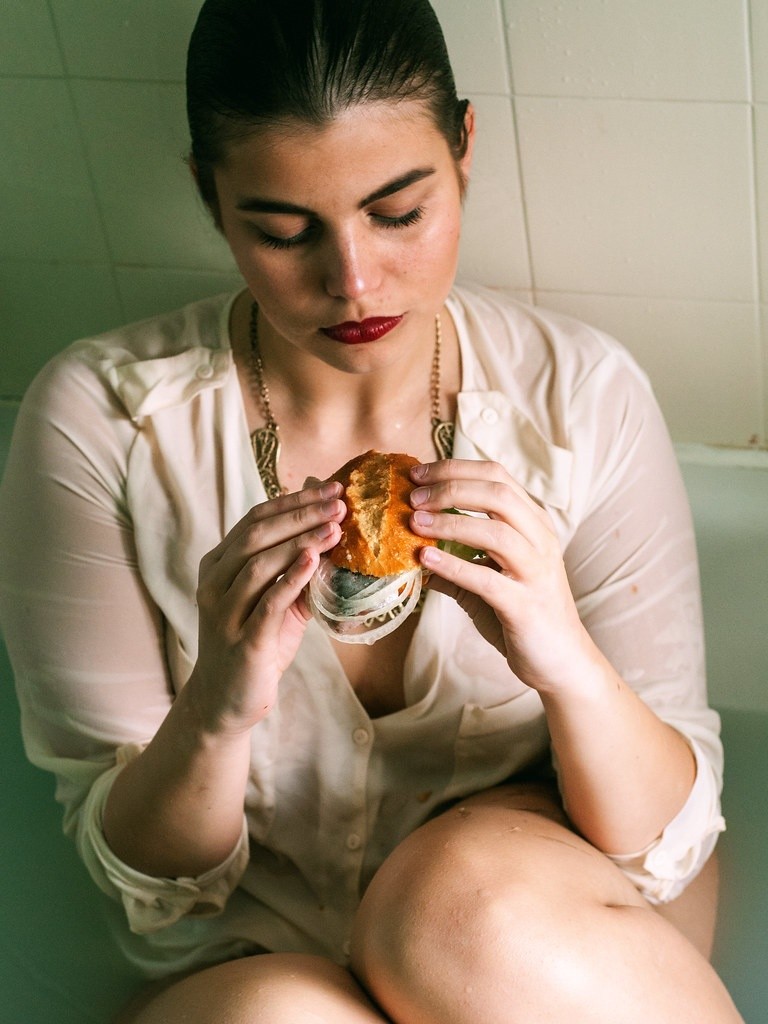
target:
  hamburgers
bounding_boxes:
[303,448,490,646]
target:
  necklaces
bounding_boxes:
[247,305,456,632]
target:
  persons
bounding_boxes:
[0,0,747,1024]
